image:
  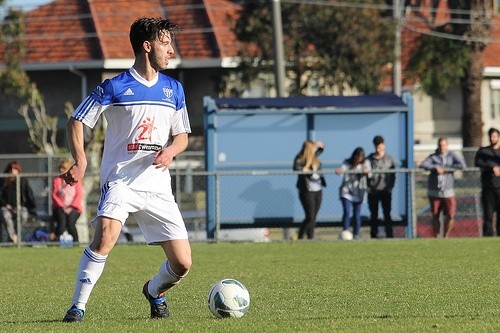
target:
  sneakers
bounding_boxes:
[63,304,84,321]
[142,280,170,319]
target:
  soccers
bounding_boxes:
[208,279,250,319]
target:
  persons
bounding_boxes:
[335,135,396,238]
[0,155,83,247]
[420,137,466,238]
[58,18,192,322]
[292,141,327,238]
[474,129,500,237]
